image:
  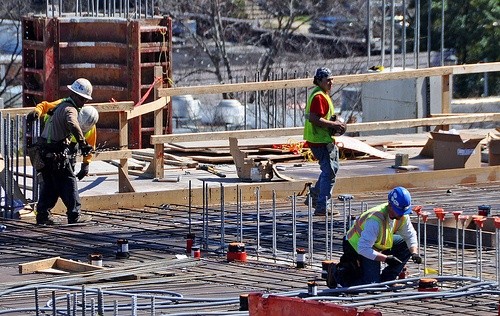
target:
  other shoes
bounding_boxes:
[37,216,62,224]
[304,198,331,210]
[380,274,400,285]
[69,215,92,223]
[327,263,339,289]
[314,210,339,215]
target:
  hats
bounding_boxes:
[316,66,332,79]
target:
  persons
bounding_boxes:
[342,187,423,291]
[27,97,99,181]
[36,78,97,227]
[303,66,345,216]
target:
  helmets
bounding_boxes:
[78,106,99,133]
[388,186,411,215]
[67,78,92,100]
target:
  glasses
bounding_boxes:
[78,95,89,103]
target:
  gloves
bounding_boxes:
[411,253,422,265]
[81,142,93,157]
[75,163,89,180]
[27,109,40,122]
[385,255,403,268]
